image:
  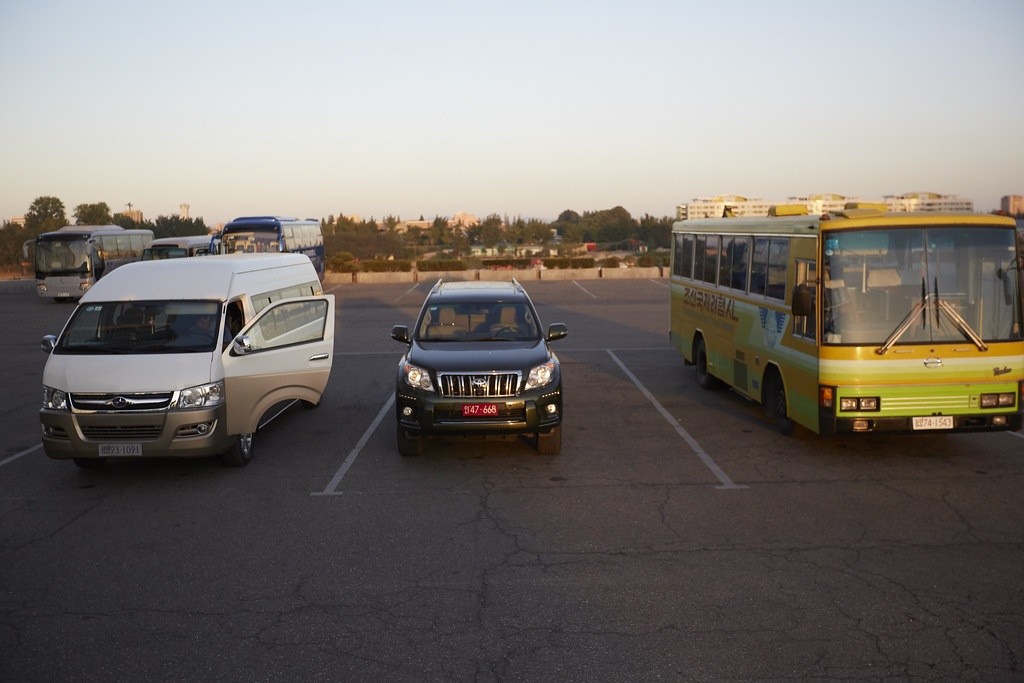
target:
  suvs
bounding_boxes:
[391,277,569,454]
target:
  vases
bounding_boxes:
[479,268,540,281]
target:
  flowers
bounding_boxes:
[481,257,544,270]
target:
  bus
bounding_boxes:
[667,201,1024,436]
[22,224,155,304]
[210,215,326,283]
[140,234,221,261]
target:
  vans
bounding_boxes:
[38,253,335,470]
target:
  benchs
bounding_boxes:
[454,314,487,331]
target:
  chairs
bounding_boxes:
[235,236,278,253]
[767,266,926,324]
[52,261,62,268]
[118,308,144,328]
[490,307,518,334]
[430,307,468,335]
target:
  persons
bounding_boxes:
[188,314,232,343]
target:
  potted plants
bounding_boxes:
[325,258,479,284]
[541,253,670,280]
